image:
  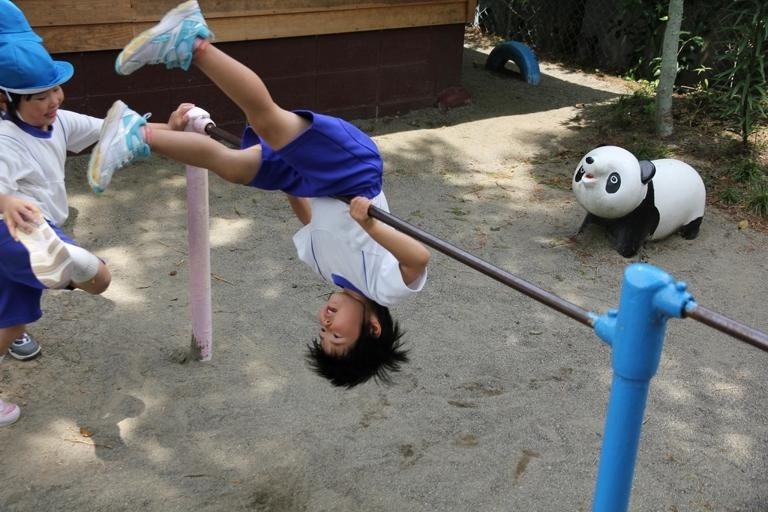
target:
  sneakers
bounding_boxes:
[0,399,20,426]
[9,331,42,360]
[87,100,151,192]
[115,0,211,76]
[17,210,74,290]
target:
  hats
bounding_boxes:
[0,0,74,94]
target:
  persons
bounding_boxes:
[0,36,197,432]
[86,0,431,391]
[1,2,47,361]
